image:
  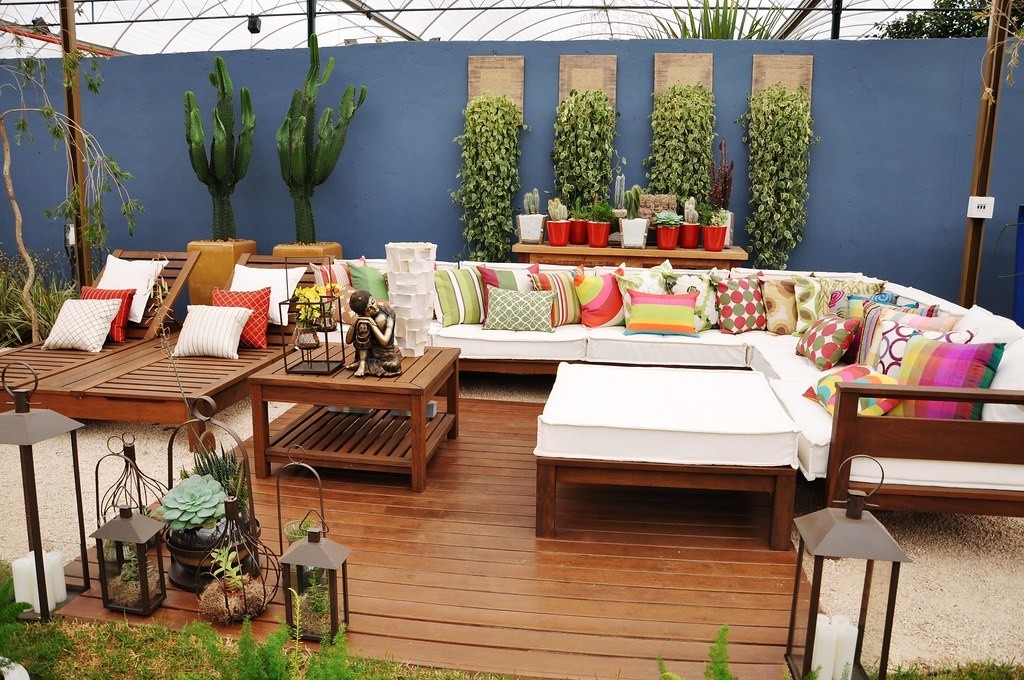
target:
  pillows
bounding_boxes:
[211,286,271,350]
[174,304,254,360]
[98,254,169,323]
[40,299,122,352]
[85,285,137,344]
[336,262,1007,417]
[229,264,308,325]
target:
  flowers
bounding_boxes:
[294,283,341,328]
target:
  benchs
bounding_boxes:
[532,361,799,552]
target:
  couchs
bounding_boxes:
[322,257,1024,523]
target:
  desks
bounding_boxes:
[512,242,748,270]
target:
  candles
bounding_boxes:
[12,550,66,613]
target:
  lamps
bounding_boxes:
[784,453,912,680]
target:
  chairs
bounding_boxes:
[0,251,200,412]
[14,254,335,426]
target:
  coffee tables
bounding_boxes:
[246,341,461,492]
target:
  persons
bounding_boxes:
[346,290,402,377]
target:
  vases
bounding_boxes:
[296,309,337,349]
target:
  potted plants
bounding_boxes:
[588,198,613,247]
[571,197,588,244]
[516,188,547,245]
[91,396,351,646]
[274,33,368,289]
[546,199,571,248]
[610,138,733,251]
[182,56,257,297]
